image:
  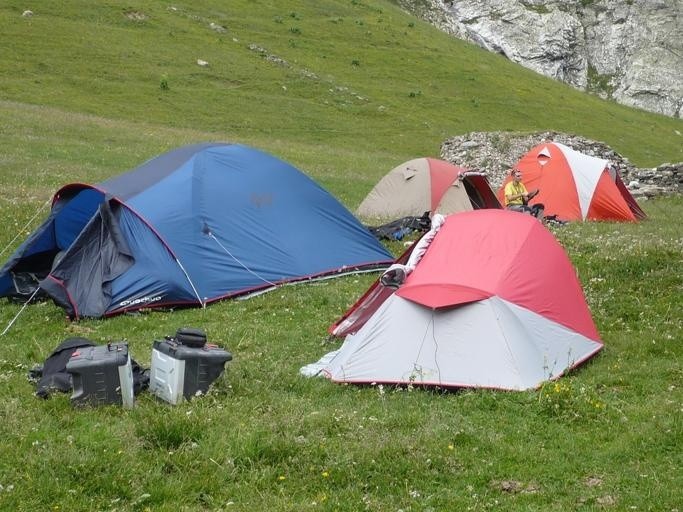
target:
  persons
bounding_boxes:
[504,168,545,222]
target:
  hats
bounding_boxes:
[511,168,522,177]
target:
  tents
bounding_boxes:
[0,141,395,337]
[352,156,501,228]
[302,209,602,391]
[495,140,646,221]
